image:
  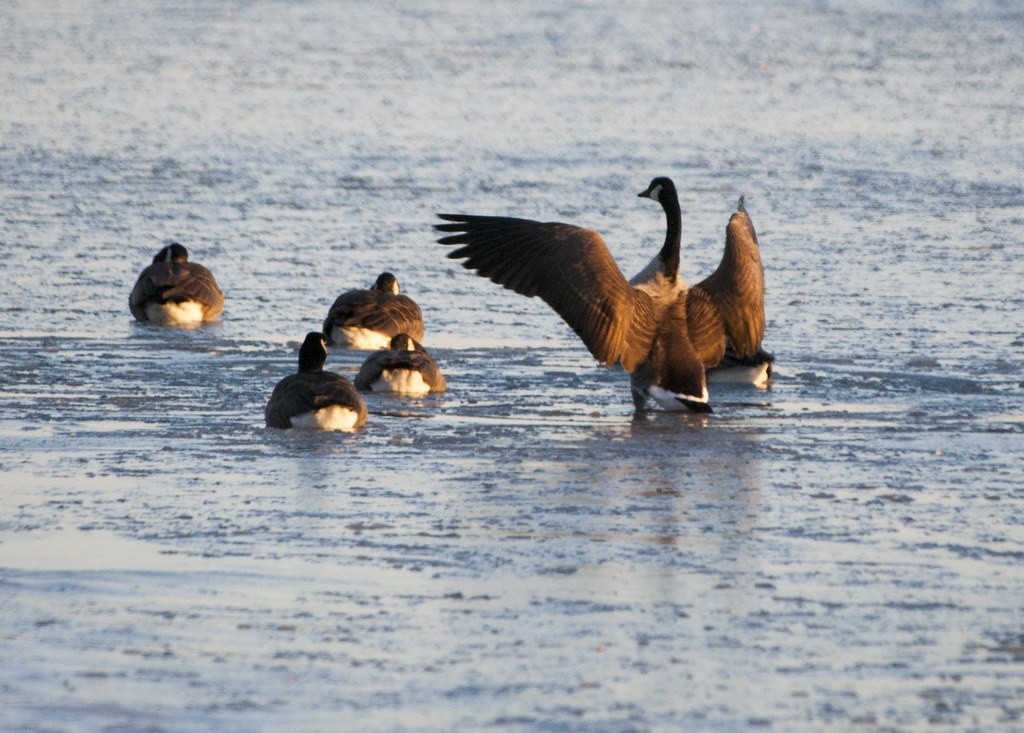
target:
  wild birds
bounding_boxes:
[265,331,368,432]
[320,272,425,350]
[431,177,767,414]
[707,347,772,389]
[353,333,449,393]
[128,242,223,322]
